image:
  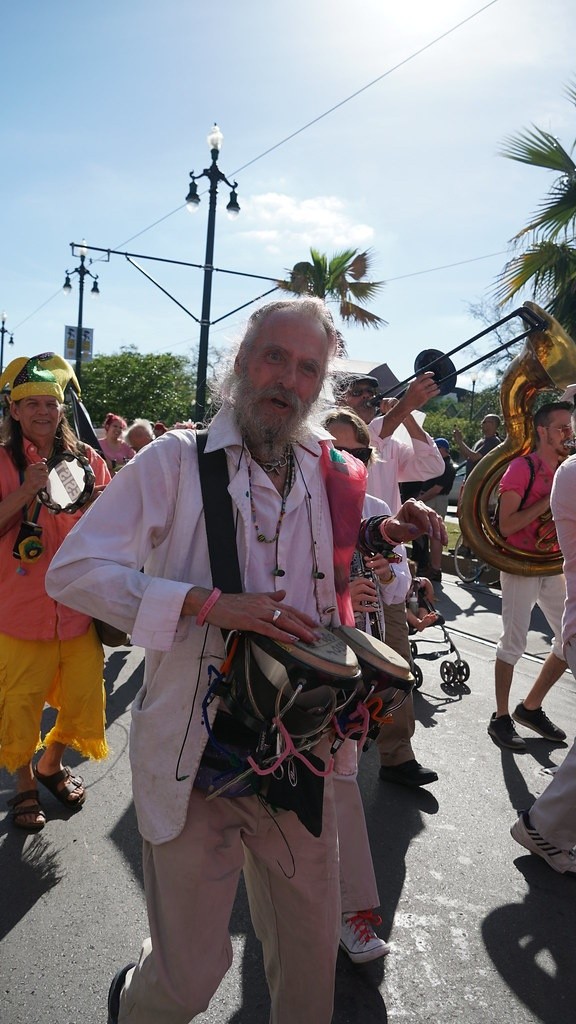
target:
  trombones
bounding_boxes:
[364,306,549,419]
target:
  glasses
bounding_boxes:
[545,426,573,438]
[350,386,378,397]
[338,446,372,463]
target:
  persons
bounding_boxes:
[317,408,412,963]
[44,296,450,1024]
[510,452,576,875]
[0,352,167,829]
[96,412,197,472]
[400,437,455,632]
[331,371,445,785]
[487,396,576,748]
[448,414,501,559]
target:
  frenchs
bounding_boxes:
[458,301,576,576]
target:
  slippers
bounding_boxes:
[7,790,47,831]
[34,762,87,808]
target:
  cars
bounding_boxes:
[418,459,467,508]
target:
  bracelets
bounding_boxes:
[379,564,396,584]
[357,514,402,556]
[195,587,221,626]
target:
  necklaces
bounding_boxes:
[248,440,325,625]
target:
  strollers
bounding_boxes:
[406,588,470,689]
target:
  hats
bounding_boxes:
[0,351,82,402]
[332,374,380,399]
[435,438,450,448]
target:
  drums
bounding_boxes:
[331,624,415,745]
[218,617,362,743]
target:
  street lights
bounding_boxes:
[0,311,14,377]
[184,121,241,428]
[62,237,100,387]
[470,370,478,422]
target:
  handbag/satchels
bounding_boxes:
[491,455,534,549]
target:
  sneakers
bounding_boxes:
[511,698,566,741]
[339,909,389,964]
[486,711,528,750]
[509,809,576,876]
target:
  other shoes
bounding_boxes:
[378,759,439,787]
[105,962,138,1024]
[417,564,441,582]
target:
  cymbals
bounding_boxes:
[35,451,96,516]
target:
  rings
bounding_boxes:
[273,609,281,624]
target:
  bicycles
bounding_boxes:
[454,517,503,587]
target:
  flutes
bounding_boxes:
[358,544,384,642]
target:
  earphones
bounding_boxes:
[273,568,286,578]
[314,572,325,579]
